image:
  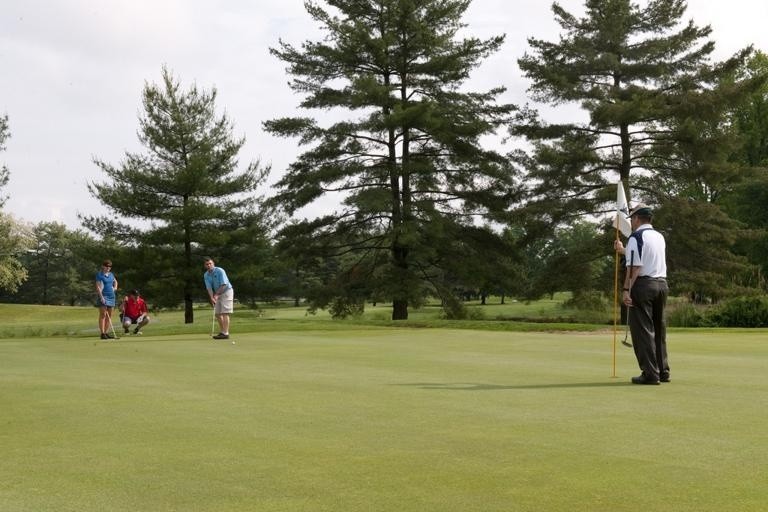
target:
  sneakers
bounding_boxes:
[135,328,142,335]
[213,333,229,339]
[632,374,671,385]
[101,333,114,339]
[124,329,129,333]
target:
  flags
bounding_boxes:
[612,180,633,239]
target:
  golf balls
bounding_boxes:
[232,342,235,345]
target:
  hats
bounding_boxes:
[626,204,651,219]
[132,289,140,296]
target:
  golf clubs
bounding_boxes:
[622,250,635,347]
[106,311,120,339]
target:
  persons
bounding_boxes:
[118,290,151,336]
[202,256,234,339]
[613,204,672,385]
[96,260,118,339]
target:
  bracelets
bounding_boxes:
[623,288,629,291]
